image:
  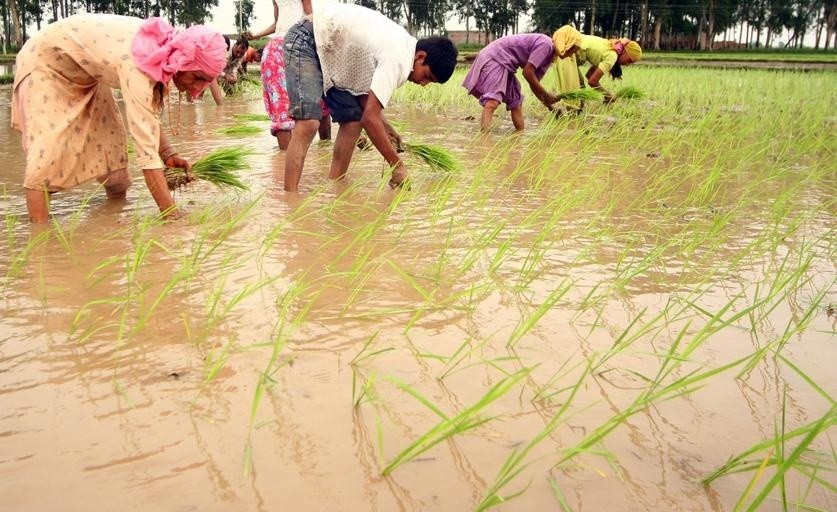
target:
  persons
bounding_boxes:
[239,0,333,153]
[216,35,250,96]
[241,45,265,82]
[280,2,460,192]
[462,22,585,133]
[554,33,642,116]
[185,34,230,107]
[7,12,231,224]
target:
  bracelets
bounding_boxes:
[157,142,178,163]
